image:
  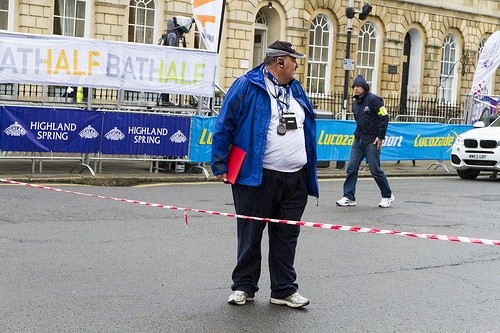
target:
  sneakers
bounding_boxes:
[378,195,395,208]
[336,197,356,207]
[228,288,256,306]
[270,291,310,309]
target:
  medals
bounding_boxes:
[277,124,288,136]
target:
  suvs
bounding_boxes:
[449,115,500,180]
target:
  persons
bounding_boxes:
[336,74,394,208]
[211,40,320,308]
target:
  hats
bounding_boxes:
[265,40,307,59]
[351,74,370,92]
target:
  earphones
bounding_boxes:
[279,60,284,65]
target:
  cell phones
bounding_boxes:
[277,124,286,135]
